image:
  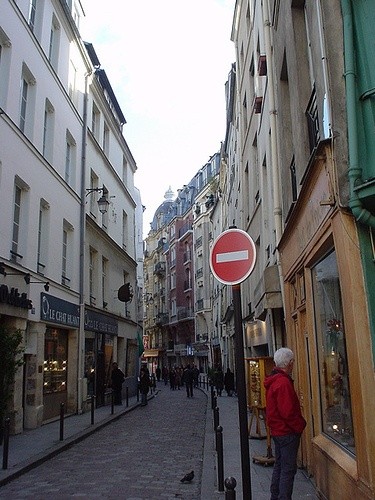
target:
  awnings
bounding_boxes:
[144,350,158,357]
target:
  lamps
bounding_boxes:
[86,187,109,215]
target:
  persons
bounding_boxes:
[161,364,199,390]
[155,366,161,382]
[140,367,151,407]
[215,367,224,397]
[182,365,196,398]
[139,363,150,380]
[264,347,306,500]
[110,362,124,406]
[224,368,235,397]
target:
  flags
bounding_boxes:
[137,333,145,358]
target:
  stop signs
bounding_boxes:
[209,228,257,286]
